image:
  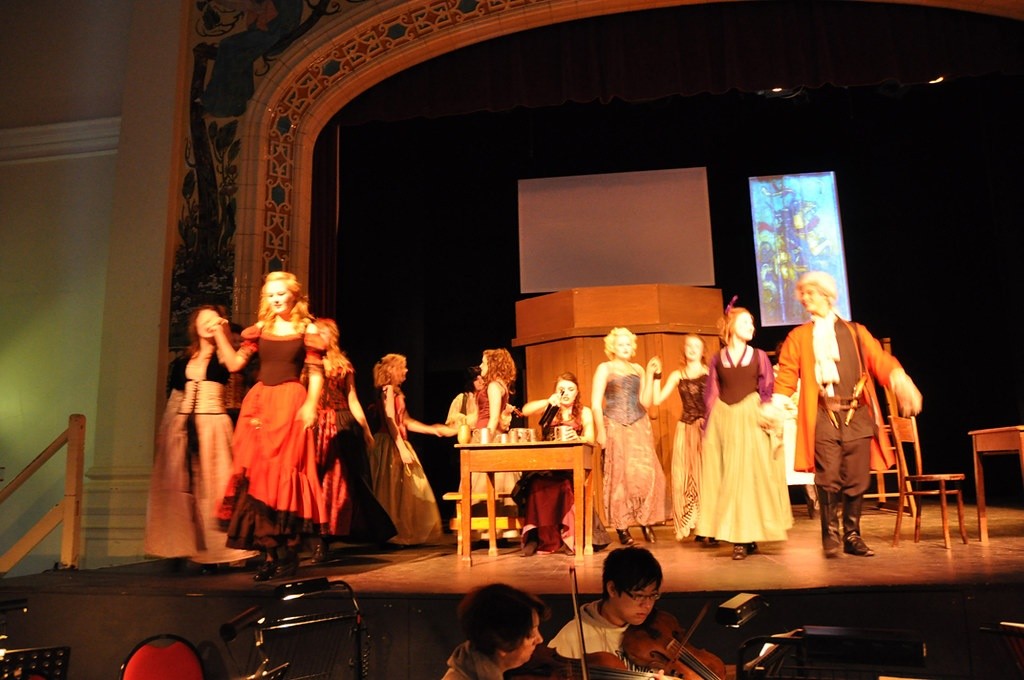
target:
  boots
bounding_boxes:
[843,494,874,557]
[818,491,840,557]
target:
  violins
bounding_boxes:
[622,608,727,680]
[503,644,684,680]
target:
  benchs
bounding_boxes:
[443,492,525,556]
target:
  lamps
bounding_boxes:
[220,605,269,667]
[273,577,363,680]
[0,599,28,626]
[714,593,772,630]
[736,626,927,680]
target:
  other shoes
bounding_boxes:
[617,529,633,545]
[732,540,756,560]
[643,524,656,541]
[695,535,717,542]
[312,544,325,563]
[254,550,297,581]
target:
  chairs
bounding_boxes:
[118,634,206,680]
[887,415,969,548]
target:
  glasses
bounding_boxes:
[626,591,661,605]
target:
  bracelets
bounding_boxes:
[653,372,661,380]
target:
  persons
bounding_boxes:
[654,333,711,544]
[141,304,261,575]
[436,348,516,548]
[694,295,795,560]
[547,545,665,680]
[511,372,596,558]
[445,366,484,418]
[763,270,923,560]
[366,354,463,546]
[299,318,399,568]
[205,270,329,582]
[590,326,675,547]
[441,583,548,680]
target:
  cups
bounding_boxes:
[457,424,471,444]
[554,426,571,442]
[473,427,494,444]
[495,428,537,444]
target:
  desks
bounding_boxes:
[454,441,598,559]
[968,426,1024,541]
